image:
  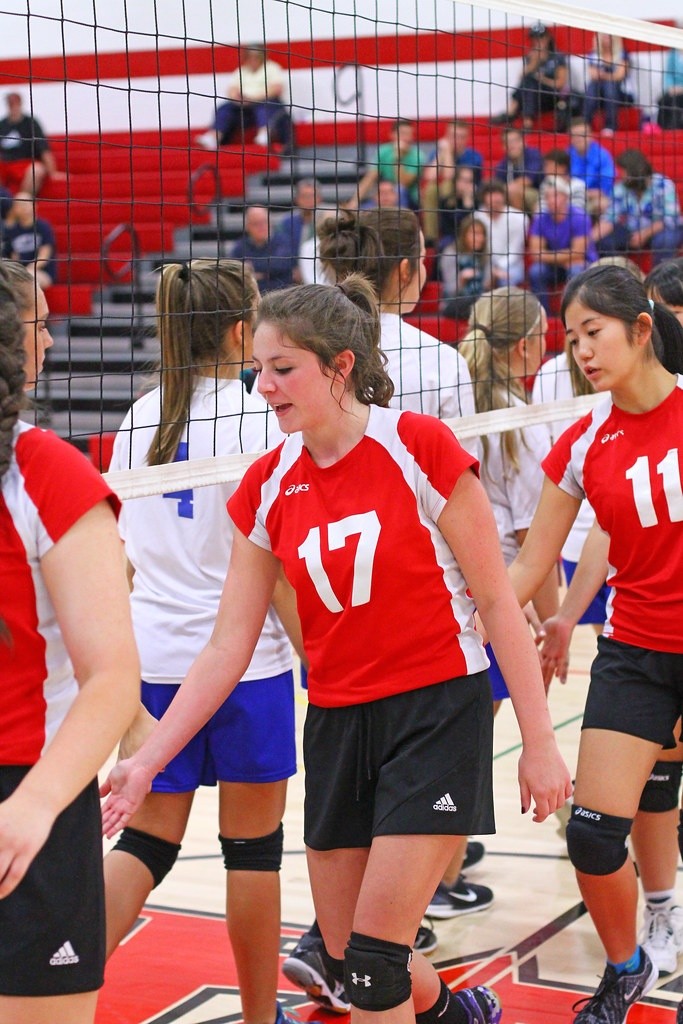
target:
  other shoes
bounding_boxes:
[455,986,502,1024]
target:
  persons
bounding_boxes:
[0,22,683,1024]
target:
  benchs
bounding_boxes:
[0,107,683,386]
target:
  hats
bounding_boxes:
[528,24,546,36]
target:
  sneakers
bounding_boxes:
[424,881,493,920]
[676,1000,683,1024]
[642,900,681,976]
[282,933,351,1013]
[411,926,437,953]
[571,946,658,1024]
[462,842,483,869]
